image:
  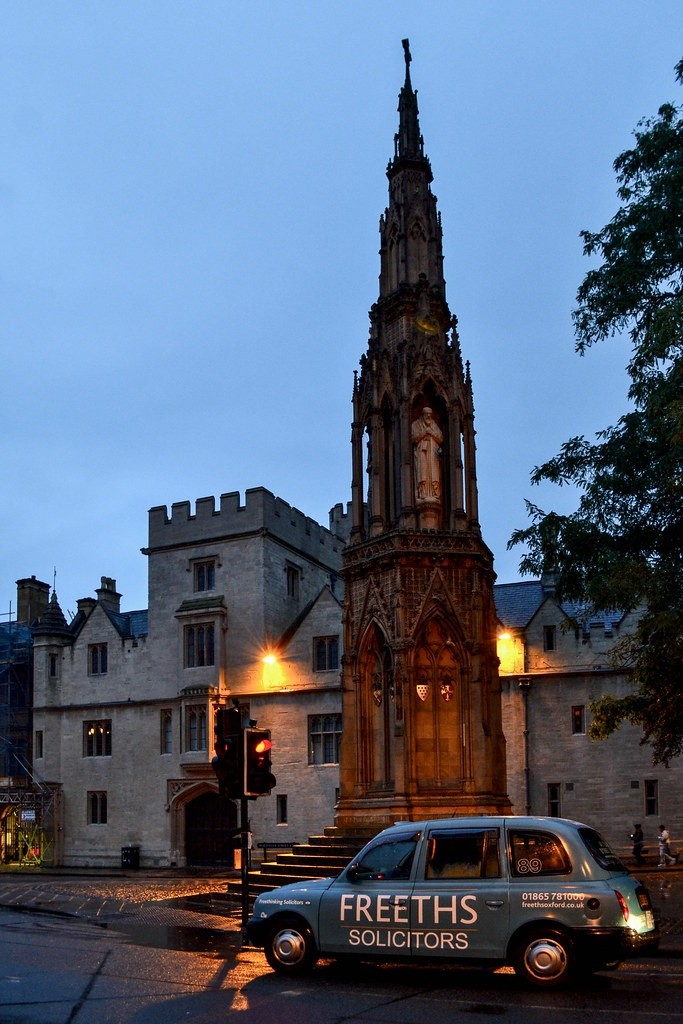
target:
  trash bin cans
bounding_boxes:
[121,846,139,869]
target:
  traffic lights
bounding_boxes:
[246,728,274,795]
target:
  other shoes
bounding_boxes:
[658,864,666,867]
[669,861,676,865]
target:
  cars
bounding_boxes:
[245,815,660,990]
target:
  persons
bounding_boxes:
[411,407,442,500]
[628,824,644,866]
[657,825,677,867]
